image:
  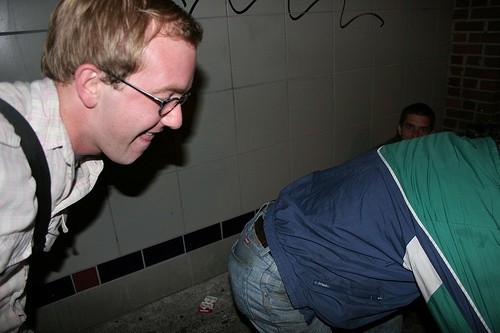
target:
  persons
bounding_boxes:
[367,102,436,151]
[225,115,500,333]
[0,0,204,333]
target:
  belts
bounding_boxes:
[256,204,276,258]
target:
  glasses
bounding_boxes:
[72,62,192,118]
